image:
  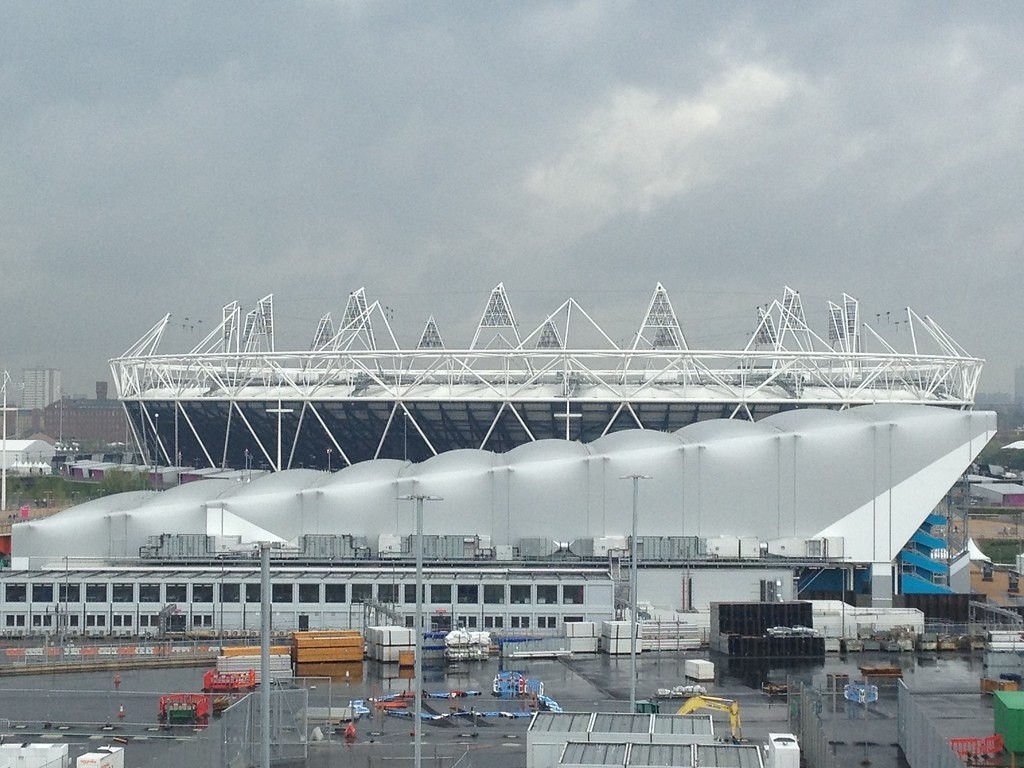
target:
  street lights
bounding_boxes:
[619,473,654,713]
[394,492,444,768]
[228,540,301,768]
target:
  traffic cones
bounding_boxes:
[117,704,126,718]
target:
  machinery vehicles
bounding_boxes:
[633,694,748,746]
